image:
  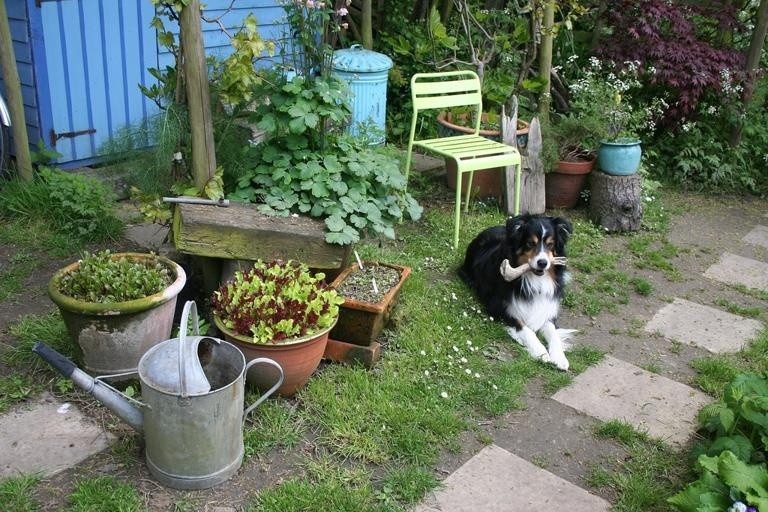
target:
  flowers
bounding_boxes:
[551,54,672,142]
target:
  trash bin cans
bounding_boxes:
[330,43,394,147]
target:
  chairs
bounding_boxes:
[399,70,522,250]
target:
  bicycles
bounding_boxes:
[0,95,13,175]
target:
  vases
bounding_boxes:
[596,137,641,176]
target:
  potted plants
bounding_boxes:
[330,258,410,346]
[46,249,186,385]
[425,1,529,198]
[210,256,345,402]
[524,110,608,209]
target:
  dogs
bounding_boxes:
[454,210,582,373]
